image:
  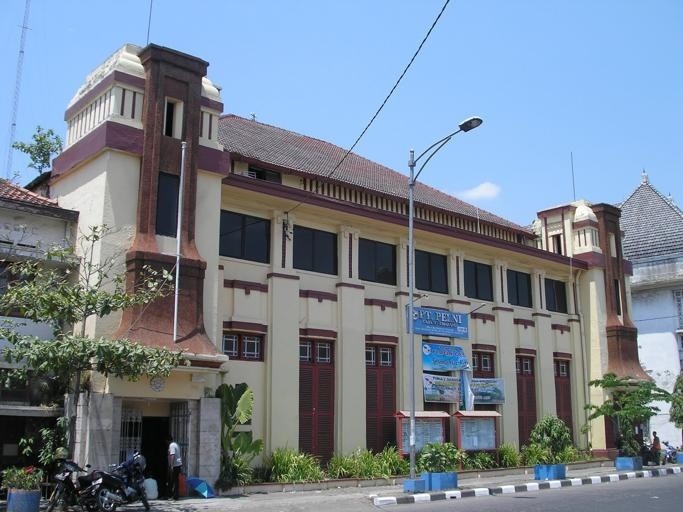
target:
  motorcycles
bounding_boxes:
[662,441,680,465]
[90,450,150,511]
[642,443,660,464]
[43,458,99,511]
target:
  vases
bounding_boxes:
[4,489,41,512]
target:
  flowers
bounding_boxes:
[2,465,40,494]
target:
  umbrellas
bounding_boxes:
[186,478,215,499]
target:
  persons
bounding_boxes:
[142,471,158,499]
[163,432,187,501]
[650,432,661,466]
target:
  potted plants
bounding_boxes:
[523,415,576,479]
[416,441,467,491]
[581,372,672,470]
[668,372,682,465]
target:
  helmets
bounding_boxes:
[133,455,146,472]
[52,446,68,461]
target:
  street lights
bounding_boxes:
[408,116,482,480]
